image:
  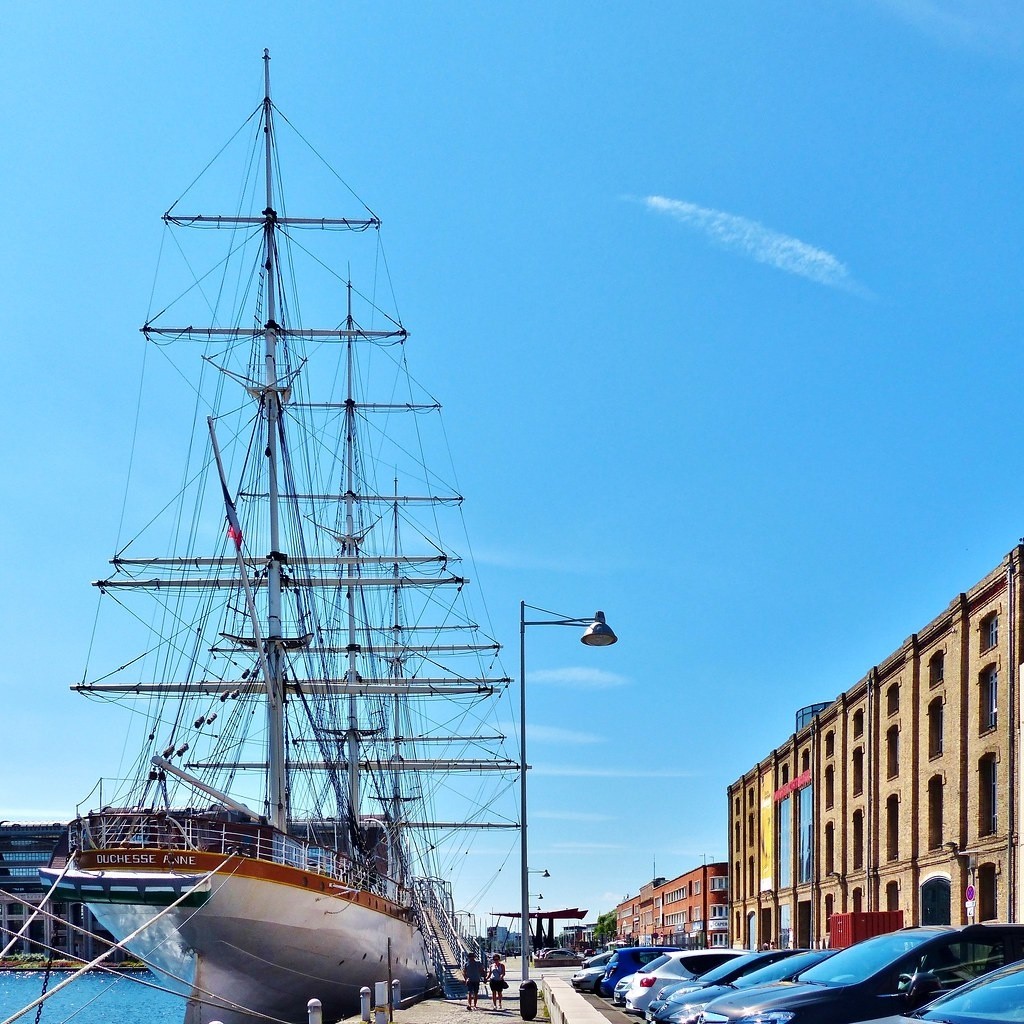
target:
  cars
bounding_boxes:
[536,945,844,1024]
[848,957,1024,1024]
[583,949,595,958]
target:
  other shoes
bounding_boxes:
[499,1005,501,1009]
[493,1006,496,1010]
[474,1007,477,1011]
[467,1005,471,1010]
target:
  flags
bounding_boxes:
[208,423,244,551]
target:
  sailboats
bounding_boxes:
[34,45,489,1024]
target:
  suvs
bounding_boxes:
[695,922,1024,1024]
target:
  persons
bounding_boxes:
[488,955,509,1011]
[461,952,487,1012]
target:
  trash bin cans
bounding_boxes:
[520,981,538,1022]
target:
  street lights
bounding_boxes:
[516,600,619,980]
